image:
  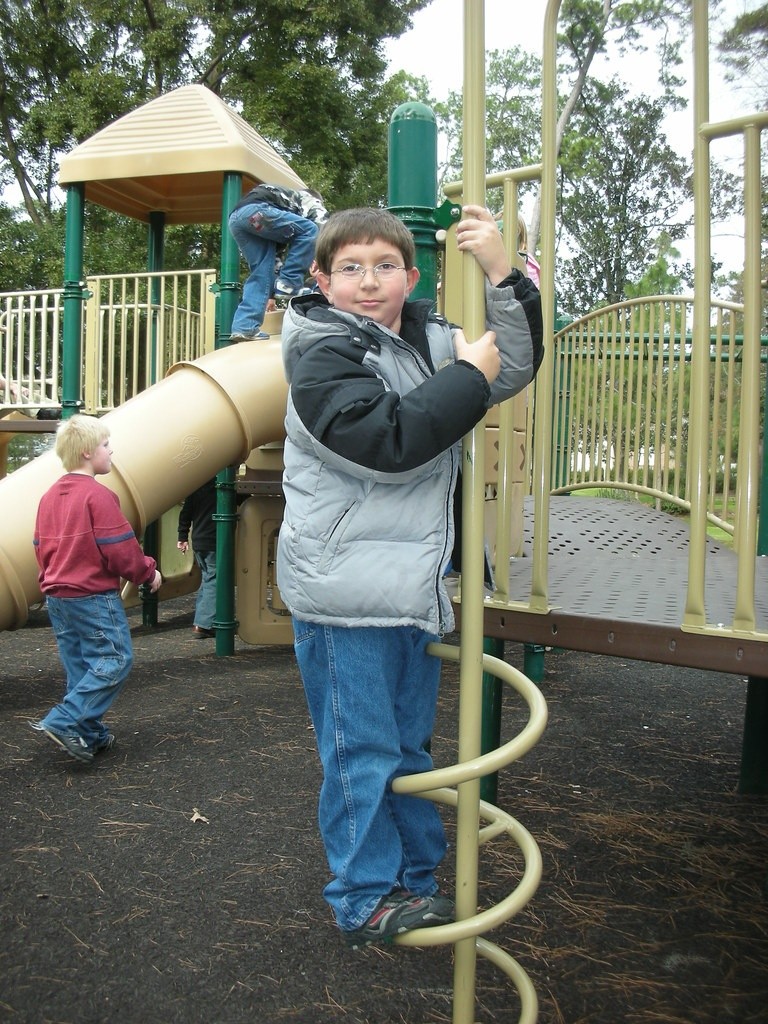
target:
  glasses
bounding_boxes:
[330,262,408,279]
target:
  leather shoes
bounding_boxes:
[191,626,215,638]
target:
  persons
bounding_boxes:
[228,184,330,342]
[178,476,253,639]
[0,373,62,420]
[437,209,540,295]
[276,201,546,948]
[33,415,162,765]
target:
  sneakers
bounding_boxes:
[273,280,322,300]
[98,734,115,748]
[39,720,95,765]
[340,891,452,941]
[228,330,269,344]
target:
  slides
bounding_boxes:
[0,333,288,630]
